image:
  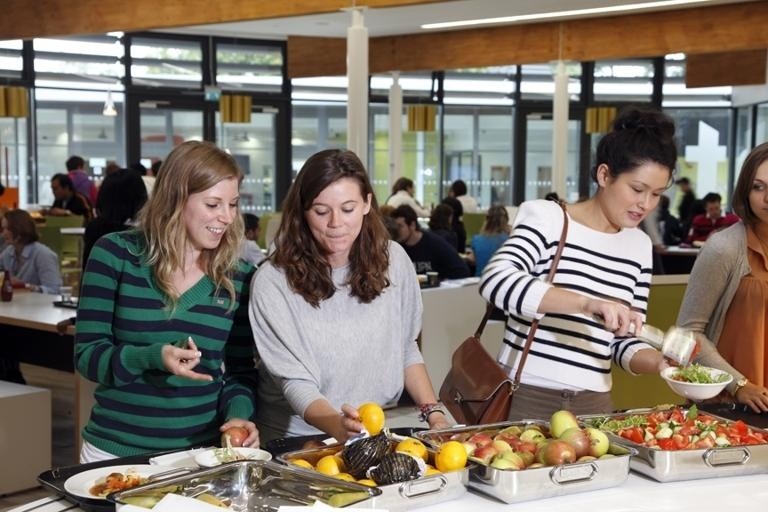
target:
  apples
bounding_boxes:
[222,427,249,447]
[468,410,622,471]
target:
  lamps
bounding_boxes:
[102,90,117,116]
[583,105,617,135]
[216,94,253,124]
[0,86,32,118]
[405,104,439,132]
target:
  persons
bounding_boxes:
[427,204,460,251]
[638,208,669,275]
[448,180,477,213]
[1,210,61,384]
[386,177,431,217]
[471,205,513,276]
[248,150,451,443]
[237,213,266,266]
[677,142,768,414]
[49,173,93,228]
[392,203,469,279]
[657,195,682,245]
[688,193,740,247]
[82,169,148,270]
[72,141,261,464]
[65,156,98,208]
[674,177,698,238]
[442,197,467,253]
[478,105,678,421]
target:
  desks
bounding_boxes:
[0,278,100,464]
[649,238,706,268]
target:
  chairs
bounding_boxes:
[257,214,285,249]
[417,287,514,400]
[40,215,84,275]
[611,283,702,408]
[462,211,486,245]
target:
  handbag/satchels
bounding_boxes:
[439,198,570,428]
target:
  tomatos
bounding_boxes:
[659,437,677,450]
[753,430,767,440]
[672,433,689,452]
[670,406,684,423]
[631,427,643,445]
[740,435,757,445]
[647,413,660,428]
[734,418,749,436]
[622,429,632,440]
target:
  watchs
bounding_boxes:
[730,377,748,398]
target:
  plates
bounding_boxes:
[149,446,273,471]
[64,464,190,501]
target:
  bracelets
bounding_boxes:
[420,402,446,422]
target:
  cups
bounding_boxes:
[58,286,73,305]
[426,272,439,287]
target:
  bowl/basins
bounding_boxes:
[659,364,734,401]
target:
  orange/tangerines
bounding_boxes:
[356,403,385,438]
[290,460,315,470]
[356,478,379,491]
[333,470,355,483]
[317,454,346,476]
[424,463,442,476]
[435,441,466,471]
[394,438,427,462]
